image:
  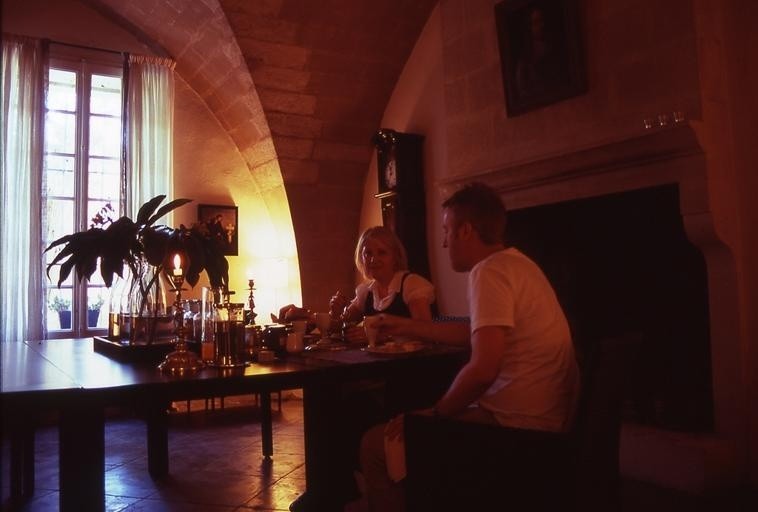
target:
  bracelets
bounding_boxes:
[431,406,442,416]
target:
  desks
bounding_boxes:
[2,329,465,512]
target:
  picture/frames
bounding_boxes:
[492,0,590,119]
[197,204,238,257]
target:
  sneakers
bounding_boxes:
[289,475,362,512]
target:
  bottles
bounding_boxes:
[178,298,217,346]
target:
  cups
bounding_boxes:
[258,350,276,362]
[286,332,304,352]
[364,314,386,346]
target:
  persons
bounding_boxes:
[362,179,580,511]
[282,218,438,511]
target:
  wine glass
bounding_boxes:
[315,313,332,339]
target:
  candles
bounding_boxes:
[172,253,183,276]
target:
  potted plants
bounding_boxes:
[41,194,230,346]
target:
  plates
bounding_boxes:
[361,339,427,354]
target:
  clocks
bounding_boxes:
[369,126,430,285]
